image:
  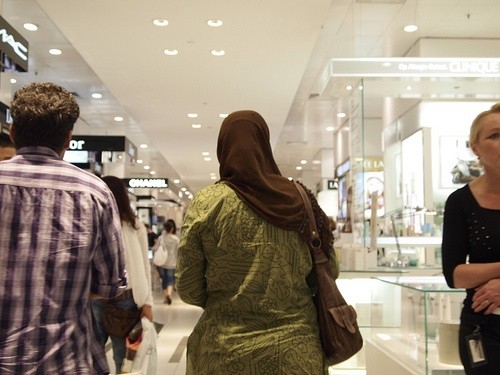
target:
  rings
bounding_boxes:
[485,300,490,305]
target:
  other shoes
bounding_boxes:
[166,295,171,304]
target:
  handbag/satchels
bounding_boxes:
[293,179,364,367]
[102,303,139,338]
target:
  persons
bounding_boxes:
[145,219,179,305]
[341,185,352,232]
[0,82,128,375]
[91,176,154,374]
[174,110,338,375]
[442,102,500,375]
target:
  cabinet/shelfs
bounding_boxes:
[352,206,445,275]
